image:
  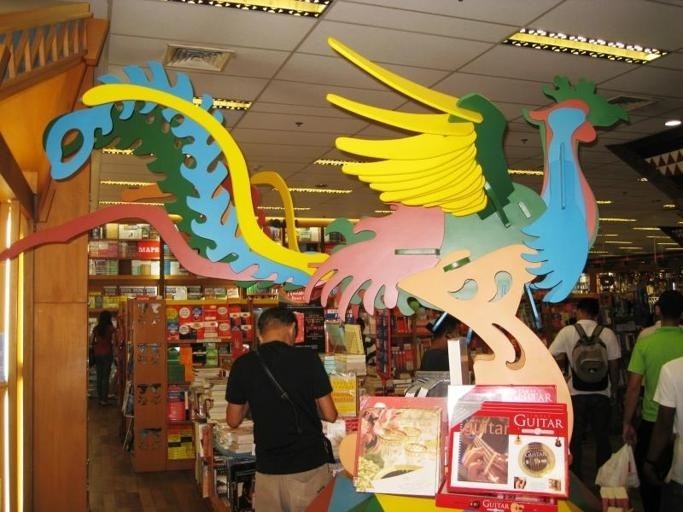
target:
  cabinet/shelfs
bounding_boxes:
[132,298,421,470]
[87,276,248,399]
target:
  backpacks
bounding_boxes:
[572,323,610,392]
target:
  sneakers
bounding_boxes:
[98,403,112,407]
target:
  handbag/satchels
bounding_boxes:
[89,348,96,370]
[322,438,336,464]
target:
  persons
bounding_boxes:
[420,316,459,371]
[356,318,377,366]
[548,290,683,512]
[89,311,119,406]
[224,307,338,512]
